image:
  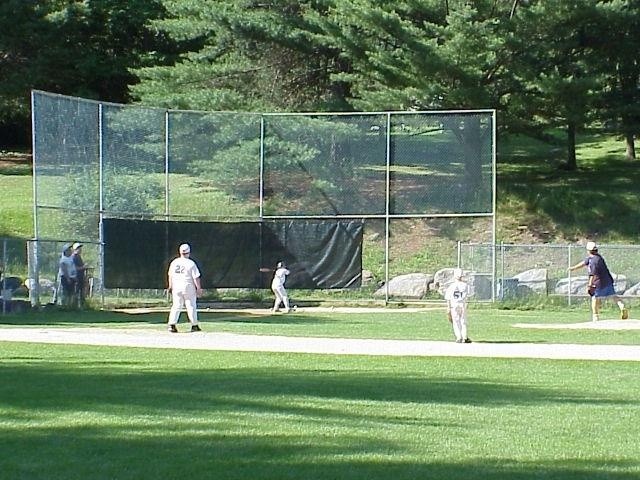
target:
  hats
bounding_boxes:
[586,241,597,251]
[61,243,71,251]
[277,261,287,268]
[72,242,82,250]
[454,269,463,277]
[179,243,190,254]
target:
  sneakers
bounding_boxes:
[456,337,471,343]
[167,325,177,332]
[191,325,200,333]
[592,314,598,321]
[621,309,628,319]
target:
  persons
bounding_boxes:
[568,241,628,322]
[168,243,201,333]
[58,243,78,311]
[69,242,89,311]
[443,268,472,343]
[271,261,296,312]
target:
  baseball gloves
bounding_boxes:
[448,312,454,324]
[588,285,596,296]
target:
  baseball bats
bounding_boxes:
[260,268,276,272]
